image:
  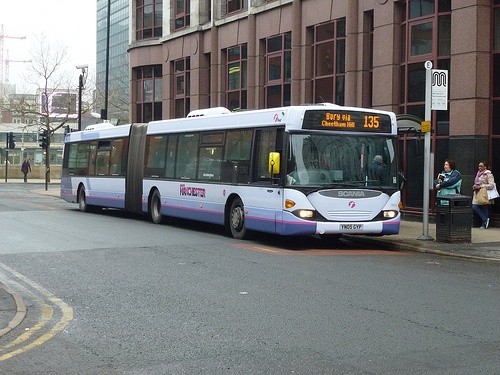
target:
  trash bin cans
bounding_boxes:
[436,194,472,244]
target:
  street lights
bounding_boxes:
[75,64,89,131]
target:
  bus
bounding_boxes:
[60,102,424,241]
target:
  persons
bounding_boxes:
[21,159,31,183]
[436,159,462,212]
[471,162,495,229]
[358,155,386,185]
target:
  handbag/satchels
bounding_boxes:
[487,183,499,200]
[472,187,494,205]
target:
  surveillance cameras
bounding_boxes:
[76,64,88,69]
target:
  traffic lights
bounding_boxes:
[9,132,16,149]
[39,130,48,149]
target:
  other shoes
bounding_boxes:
[480,218,490,228]
